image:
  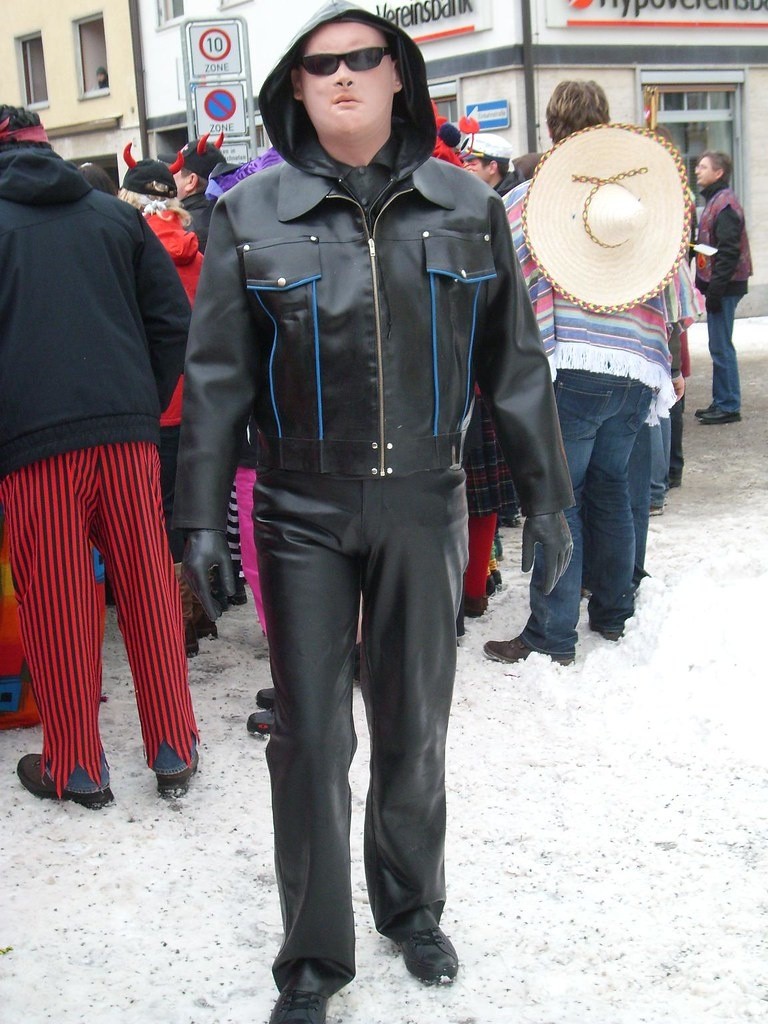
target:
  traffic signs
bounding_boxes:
[467,100,510,131]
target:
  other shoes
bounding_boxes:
[256,688,277,708]
[247,711,274,736]
[700,410,740,423]
[230,585,247,605]
[649,505,666,516]
[695,404,718,417]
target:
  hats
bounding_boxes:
[122,142,184,198]
[459,133,512,163]
[439,122,460,147]
[96,67,105,74]
[157,131,226,179]
[522,123,693,314]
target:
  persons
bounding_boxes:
[166,0,570,1020]
[96,68,109,87]
[0,81,753,812]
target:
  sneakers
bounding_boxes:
[269,990,327,1024]
[396,927,458,979]
[17,754,114,809]
[155,749,199,796]
[483,636,575,666]
[589,617,622,641]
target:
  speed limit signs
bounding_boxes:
[189,23,242,76]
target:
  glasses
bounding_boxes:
[297,47,393,76]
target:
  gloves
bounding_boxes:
[522,510,574,595]
[181,529,235,622]
[705,295,721,313]
